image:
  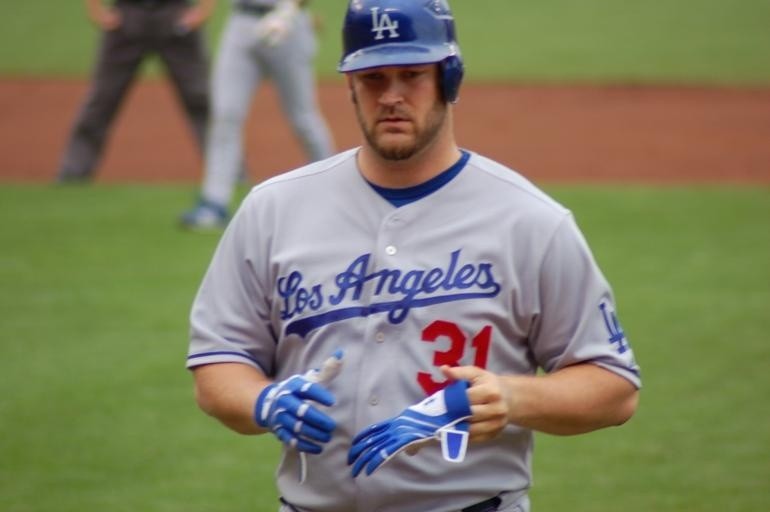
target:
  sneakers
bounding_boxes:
[174,198,231,228]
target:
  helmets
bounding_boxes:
[334,1,464,106]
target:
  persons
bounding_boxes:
[185,0,641,511]
[55,0,216,185]
[174,0,335,232]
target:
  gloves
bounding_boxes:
[254,351,344,454]
[346,379,473,480]
